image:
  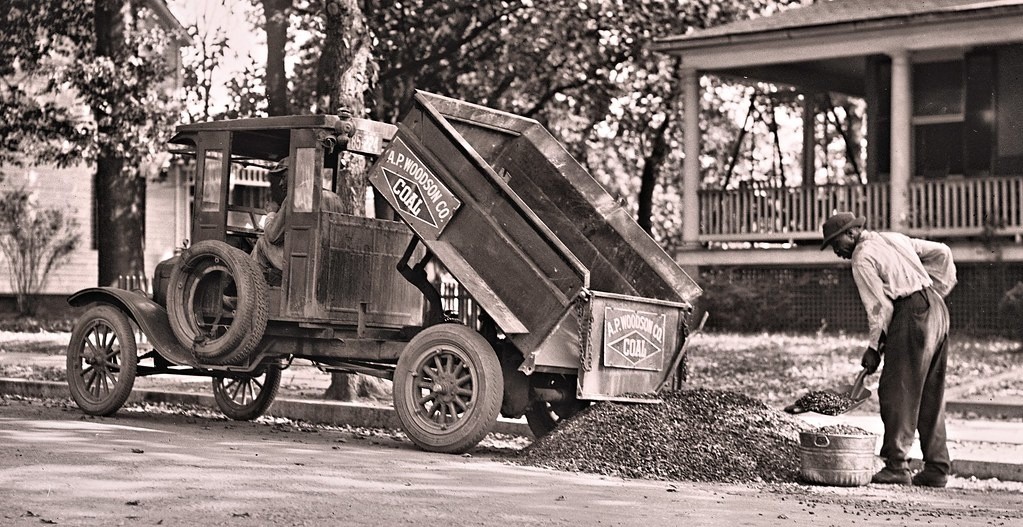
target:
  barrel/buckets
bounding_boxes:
[799,433,876,487]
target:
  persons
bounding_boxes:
[820,213,958,486]
[223,156,346,309]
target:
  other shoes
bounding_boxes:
[911,463,947,487]
[871,467,912,486]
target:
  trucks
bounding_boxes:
[65,90,705,453]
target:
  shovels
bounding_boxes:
[797,341,886,416]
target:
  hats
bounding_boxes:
[270,156,290,173]
[820,212,866,250]
